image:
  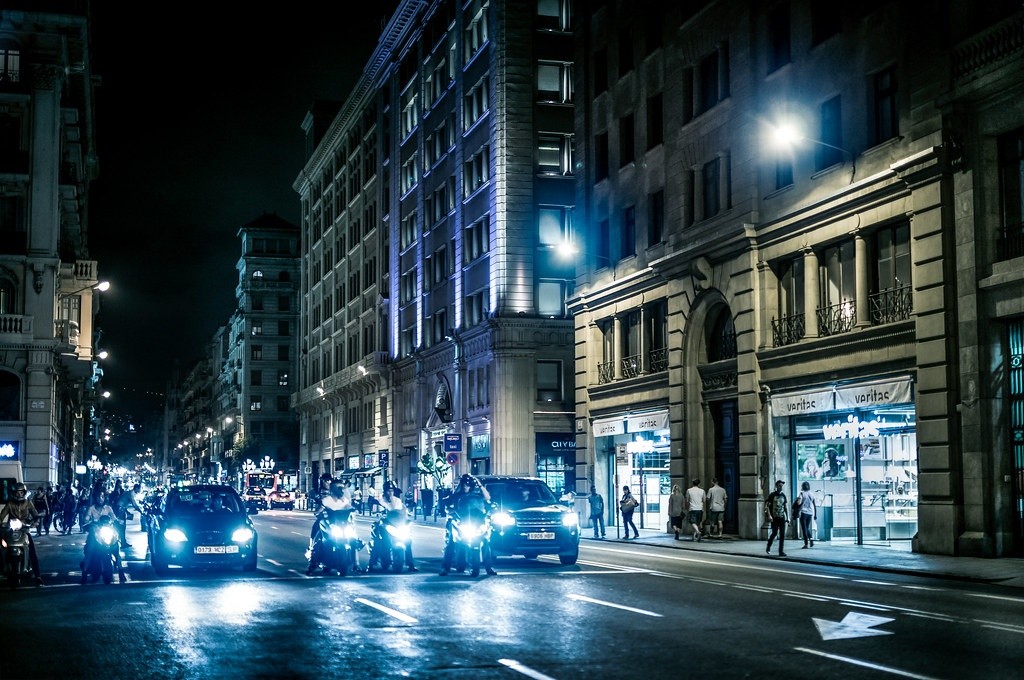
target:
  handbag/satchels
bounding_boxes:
[792,492,803,518]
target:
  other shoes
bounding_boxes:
[811,538,814,547]
[778,552,787,556]
[623,535,630,539]
[633,534,639,539]
[801,545,808,549]
[697,532,701,542]
[766,547,770,554]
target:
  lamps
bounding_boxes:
[59,280,110,301]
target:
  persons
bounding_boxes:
[668,485,686,539]
[0,482,45,587]
[204,495,233,514]
[707,478,727,538]
[620,485,640,539]
[80,491,126,584]
[791,481,818,549]
[588,486,606,539]
[686,479,707,542]
[304,473,362,574]
[363,481,420,572]
[439,475,498,576]
[765,480,790,556]
[31,478,147,548]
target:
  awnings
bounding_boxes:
[341,467,381,478]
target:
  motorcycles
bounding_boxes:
[367,495,416,574]
[439,489,499,577]
[0,513,47,589]
[79,513,125,586]
[313,495,366,578]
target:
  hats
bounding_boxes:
[775,480,785,485]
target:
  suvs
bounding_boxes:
[449,474,581,565]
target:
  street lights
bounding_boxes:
[823,415,880,545]
[625,436,656,529]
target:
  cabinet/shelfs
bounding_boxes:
[801,436,918,539]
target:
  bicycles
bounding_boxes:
[53,514,66,533]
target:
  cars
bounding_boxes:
[138,484,259,575]
[239,486,294,511]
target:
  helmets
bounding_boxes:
[383,481,394,490]
[330,481,344,498]
[10,483,27,500]
